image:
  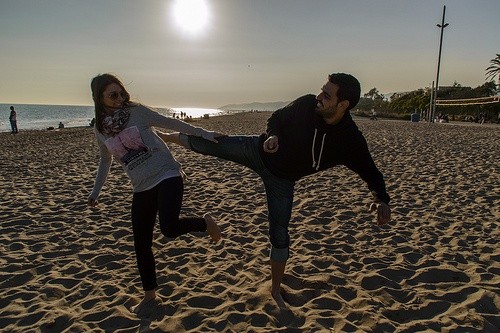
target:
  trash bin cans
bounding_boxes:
[411,113,420,122]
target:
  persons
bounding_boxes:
[87,73,228,314]
[8,106,18,134]
[173,111,192,120]
[155,73,392,310]
[373,110,377,120]
[465,108,500,125]
[439,112,449,122]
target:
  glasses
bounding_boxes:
[103,91,126,99]
